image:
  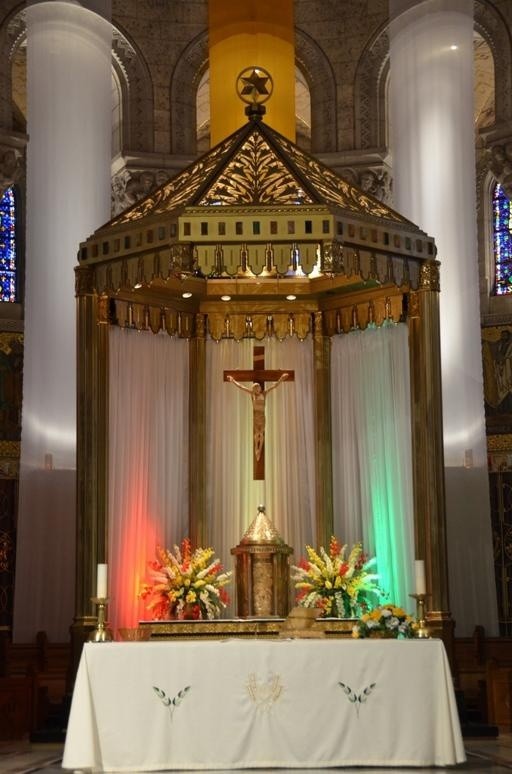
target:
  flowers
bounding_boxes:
[350,602,420,639]
[287,536,389,620]
[137,537,235,624]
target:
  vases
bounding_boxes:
[368,627,400,640]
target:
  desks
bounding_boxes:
[59,637,469,769]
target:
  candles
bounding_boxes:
[412,559,430,595]
[96,563,108,599]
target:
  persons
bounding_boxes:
[224,371,291,464]
